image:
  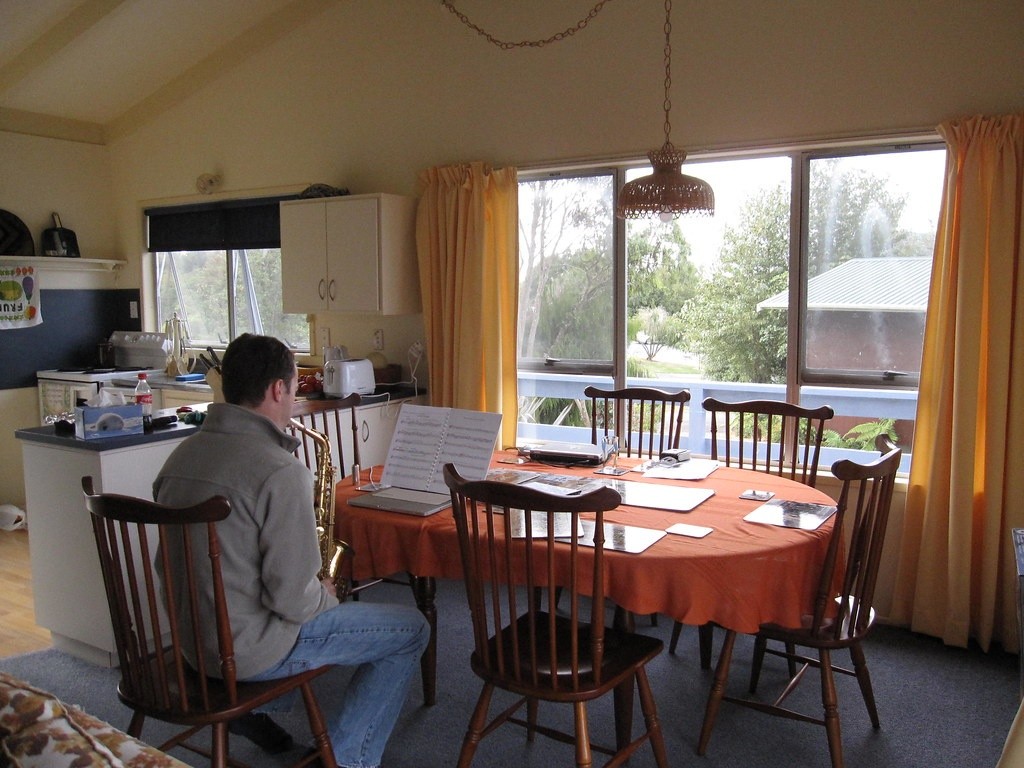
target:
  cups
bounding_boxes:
[96,342,115,369]
[601,435,619,474]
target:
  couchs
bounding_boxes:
[1,676,201,768]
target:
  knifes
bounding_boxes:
[199,346,222,375]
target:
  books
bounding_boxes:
[380,403,503,495]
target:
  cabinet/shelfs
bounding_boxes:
[287,395,428,490]
[280,191,423,314]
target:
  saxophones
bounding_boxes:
[288,415,359,602]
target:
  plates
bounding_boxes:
[94,369,116,373]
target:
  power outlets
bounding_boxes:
[373,329,383,350]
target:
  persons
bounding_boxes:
[153,332,431,768]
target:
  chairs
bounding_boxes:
[552,383,691,624]
[667,398,834,676]
[694,434,904,767]
[82,477,338,768]
[441,463,669,767]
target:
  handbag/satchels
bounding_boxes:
[0,504,27,532]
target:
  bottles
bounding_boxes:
[135,373,153,418]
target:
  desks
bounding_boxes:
[314,450,848,707]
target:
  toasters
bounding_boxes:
[322,357,376,400]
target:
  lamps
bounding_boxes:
[615,0,717,219]
[196,174,221,194]
